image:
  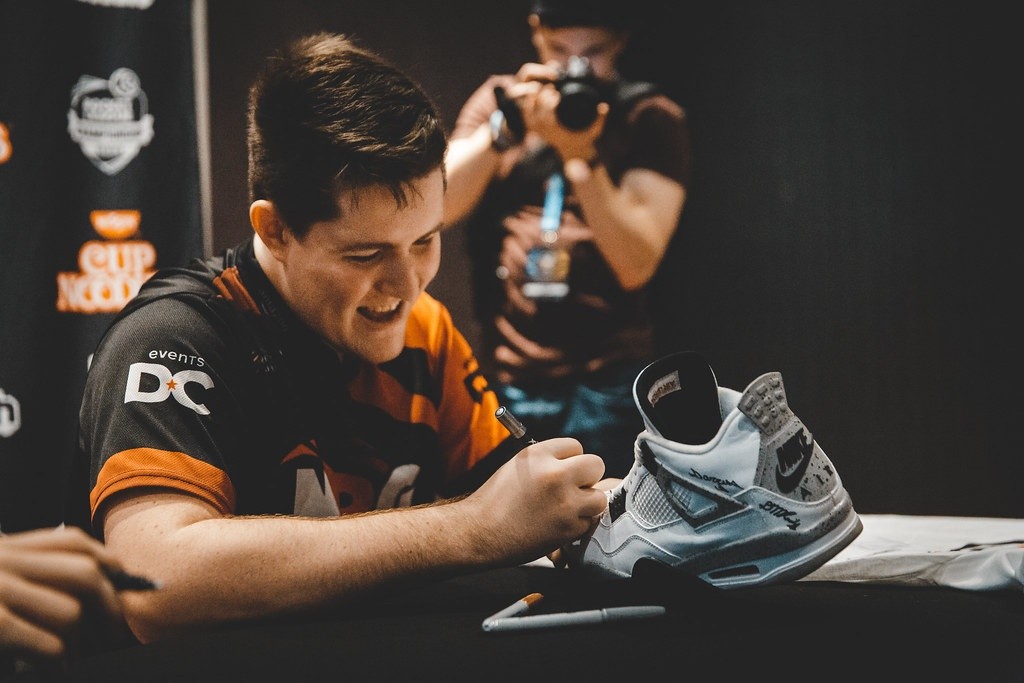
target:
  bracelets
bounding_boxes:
[564,152,601,183]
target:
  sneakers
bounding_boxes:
[551,347,863,593]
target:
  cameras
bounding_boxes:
[537,57,618,131]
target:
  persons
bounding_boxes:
[0,35,621,683]
[441,0,689,480]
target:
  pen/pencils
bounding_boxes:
[494,405,538,448]
[99,563,162,593]
